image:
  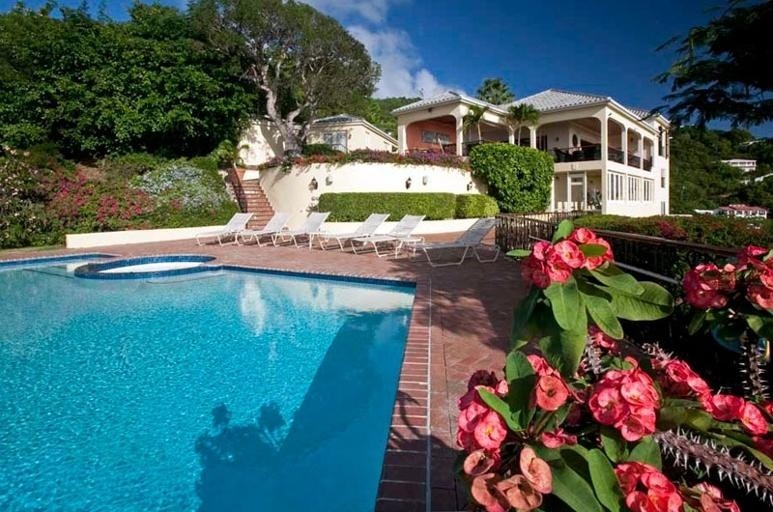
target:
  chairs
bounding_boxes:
[196,210,328,249]
[318,212,500,268]
